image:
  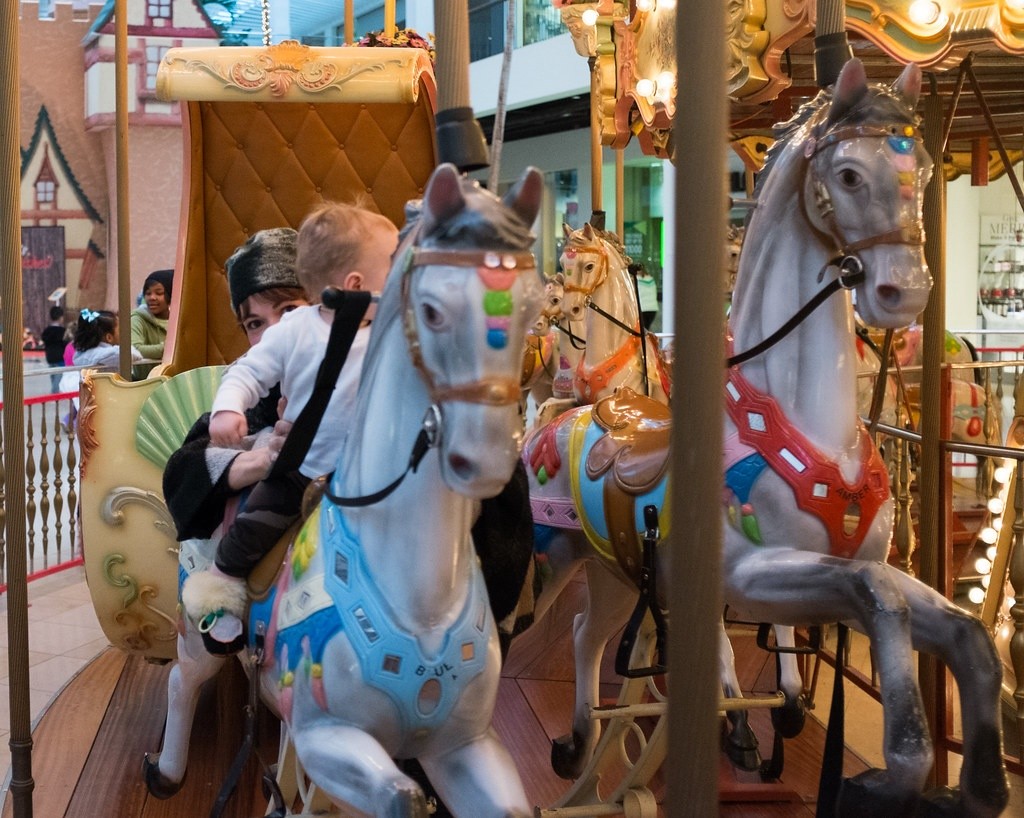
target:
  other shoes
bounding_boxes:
[61,418,70,433]
[182,570,247,657]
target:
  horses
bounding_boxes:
[526,59,1010,818]
[145,163,551,818]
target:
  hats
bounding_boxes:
[224,228,299,315]
[143,269,175,304]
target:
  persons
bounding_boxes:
[42,306,70,394]
[161,200,544,817]
[60,269,177,433]
